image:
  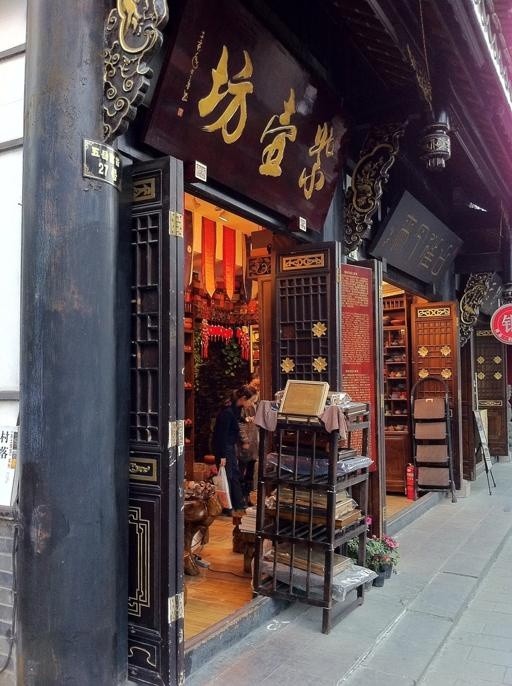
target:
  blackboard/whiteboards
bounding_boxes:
[473,410,493,472]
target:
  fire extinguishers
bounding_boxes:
[404,462,415,499]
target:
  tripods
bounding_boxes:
[475,442,496,495]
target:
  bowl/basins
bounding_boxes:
[390,320,403,326]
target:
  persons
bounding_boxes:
[210,385,259,518]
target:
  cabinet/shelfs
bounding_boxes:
[184,329,194,481]
[253,401,370,634]
[382,290,411,493]
[249,324,261,375]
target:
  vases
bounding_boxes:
[380,564,393,579]
[372,571,385,587]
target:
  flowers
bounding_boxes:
[347,535,391,574]
[380,537,403,565]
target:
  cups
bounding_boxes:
[382,315,392,326]
